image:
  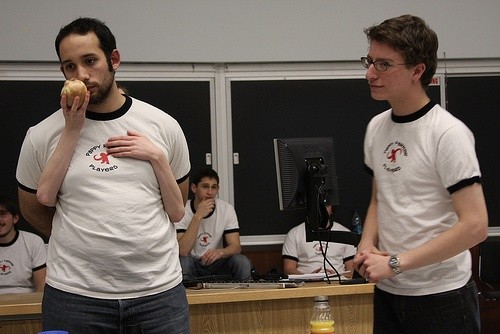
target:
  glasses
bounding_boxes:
[361,57,410,72]
[0,209,13,217]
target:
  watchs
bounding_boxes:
[389,255,401,275]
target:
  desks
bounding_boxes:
[0,278,375,334]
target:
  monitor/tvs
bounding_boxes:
[273,137,340,211]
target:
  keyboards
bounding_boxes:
[197,280,305,288]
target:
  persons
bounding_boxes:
[36,86,185,223]
[352,15,489,334]
[0,202,46,293]
[282,206,357,277]
[15,17,190,334]
[175,169,251,284]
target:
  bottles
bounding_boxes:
[310,295,334,334]
[351,210,363,235]
